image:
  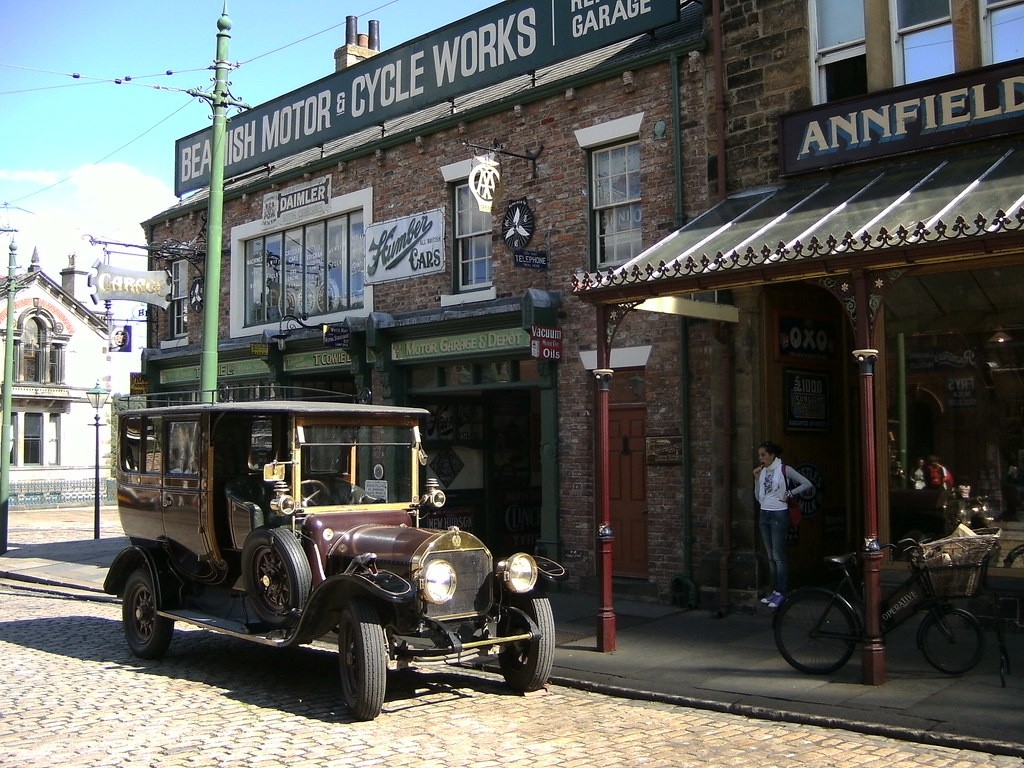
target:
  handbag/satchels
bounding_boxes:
[788,499,801,527]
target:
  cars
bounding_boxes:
[102,384,567,721]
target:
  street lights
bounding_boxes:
[86,379,113,541]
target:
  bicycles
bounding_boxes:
[770,526,1004,677]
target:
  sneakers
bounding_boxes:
[761,590,777,603]
[768,591,783,607]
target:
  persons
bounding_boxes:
[752,440,813,608]
[910,456,949,490]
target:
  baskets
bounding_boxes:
[926,558,988,598]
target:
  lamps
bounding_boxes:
[988,325,1014,342]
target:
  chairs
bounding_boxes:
[223,474,351,549]
